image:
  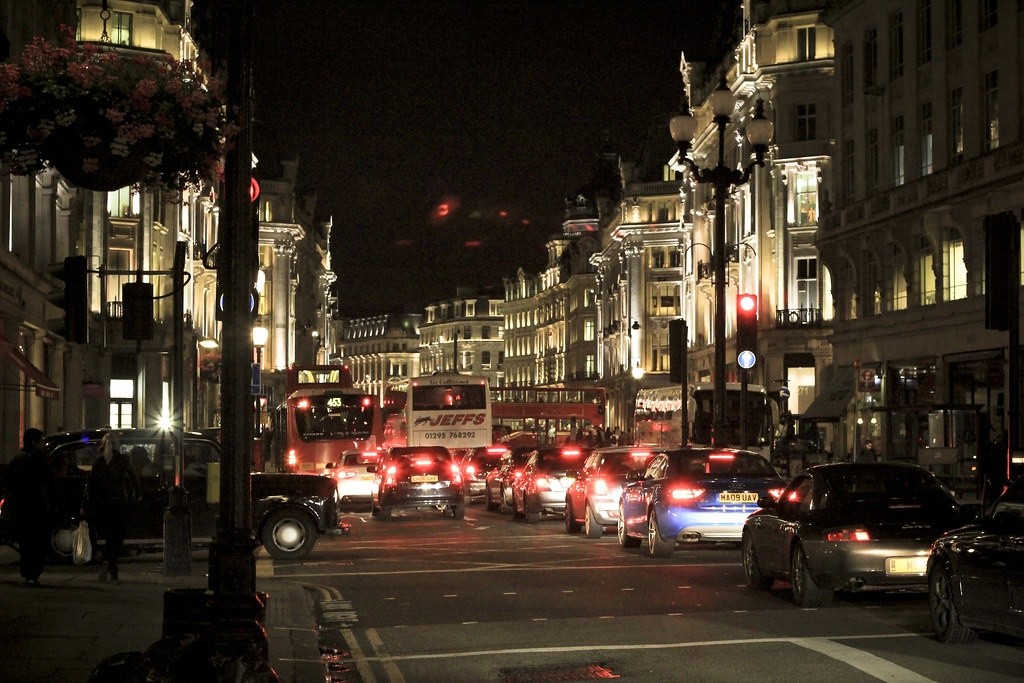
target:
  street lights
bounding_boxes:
[252,327,268,436]
[670,62,775,447]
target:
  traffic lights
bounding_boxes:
[43,255,88,344]
[220,171,260,280]
[736,294,757,367]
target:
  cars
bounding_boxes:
[741,460,976,608]
[926,474,1024,644]
[326,449,385,504]
[449,443,791,558]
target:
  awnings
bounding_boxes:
[801,381,855,423]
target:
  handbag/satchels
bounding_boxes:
[71,520,92,567]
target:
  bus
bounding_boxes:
[274,364,384,473]
[407,373,493,449]
[634,382,778,446]
[383,386,607,447]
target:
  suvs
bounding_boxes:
[379,447,467,521]
[40,427,339,561]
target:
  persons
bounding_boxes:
[576,426,634,448]
[861,440,877,463]
[132,446,153,476]
[0,428,59,586]
[548,424,556,440]
[80,431,128,584]
